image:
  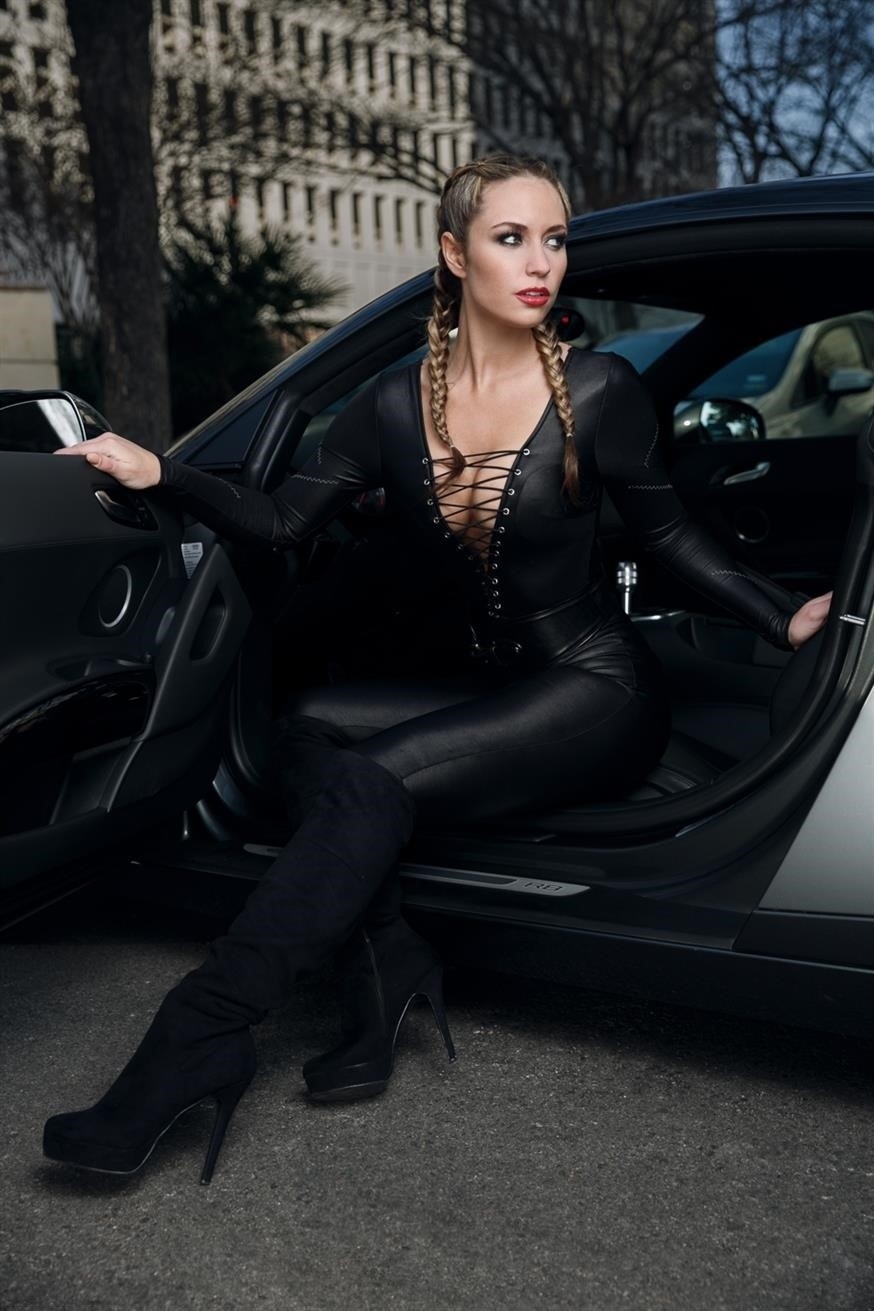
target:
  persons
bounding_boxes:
[42,152,833,1185]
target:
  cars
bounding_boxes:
[1,174,874,1007]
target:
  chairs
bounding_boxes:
[625,625,827,801]
[643,611,795,669]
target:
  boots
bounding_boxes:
[273,714,458,1102]
[42,749,419,1186]
